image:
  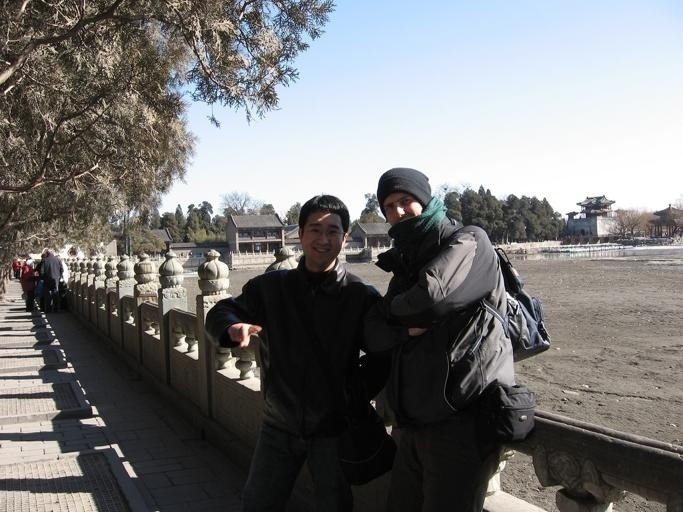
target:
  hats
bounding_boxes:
[378,167,433,209]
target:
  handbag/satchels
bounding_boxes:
[483,381,538,444]
[337,422,398,488]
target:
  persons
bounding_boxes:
[12,248,64,314]
[363,167,516,512]
[204,194,400,511]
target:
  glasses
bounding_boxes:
[306,225,347,240]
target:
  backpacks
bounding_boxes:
[495,249,552,362]
